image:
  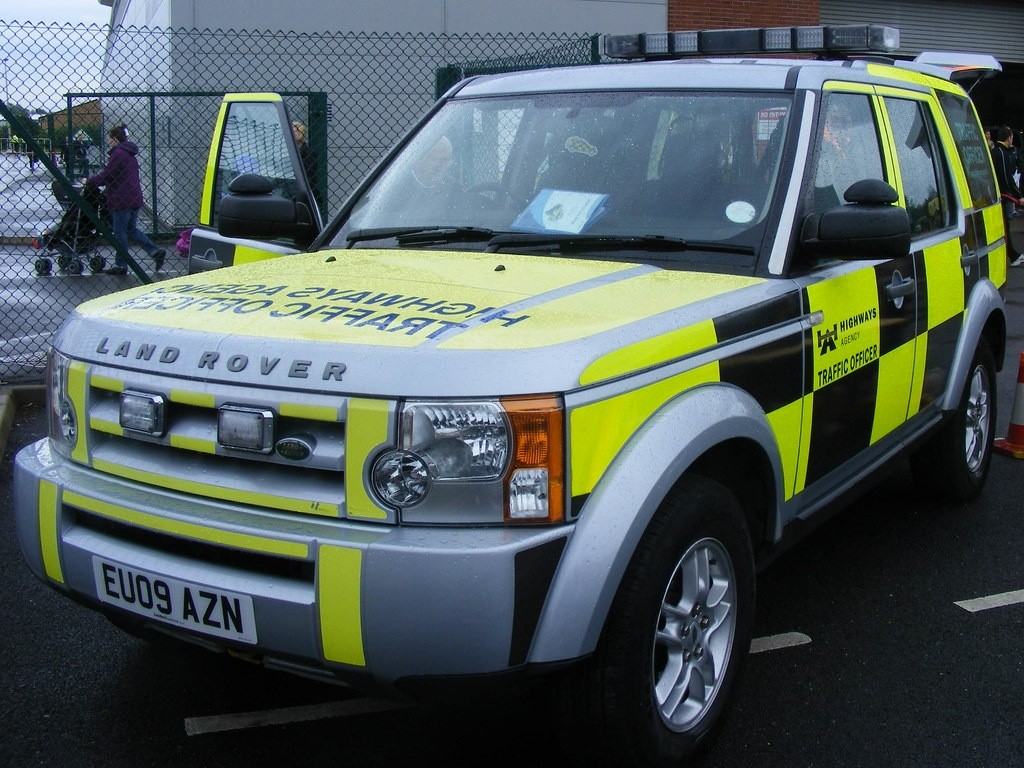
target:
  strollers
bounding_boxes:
[35,177,108,274]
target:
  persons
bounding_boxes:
[291,122,322,209]
[383,136,480,219]
[74,130,89,180]
[991,126,1024,267]
[985,128,994,149]
[81,125,166,276]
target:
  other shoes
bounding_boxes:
[154,250,166,271]
[107,265,127,275]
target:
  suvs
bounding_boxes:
[11,25,979,757]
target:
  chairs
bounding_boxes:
[536,116,788,241]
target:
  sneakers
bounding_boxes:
[1011,253,1024,267]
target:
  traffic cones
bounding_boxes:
[993,351,1024,457]
